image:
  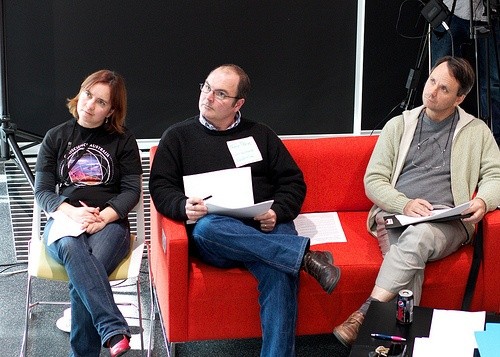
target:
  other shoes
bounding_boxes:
[107,334,130,357]
[334,312,366,349]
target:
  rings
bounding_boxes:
[265,224,266,227]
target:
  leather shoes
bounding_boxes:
[303,249,341,295]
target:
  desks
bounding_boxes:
[349,298,500,357]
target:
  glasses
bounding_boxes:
[412,137,446,169]
[199,82,242,100]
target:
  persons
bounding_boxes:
[149,63,341,357]
[33,69,144,357]
[333,56,500,347]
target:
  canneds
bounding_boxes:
[395,289,414,323]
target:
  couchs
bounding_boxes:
[146,129,500,357]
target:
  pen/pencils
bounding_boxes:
[192,195,213,205]
[79,200,100,219]
[370,334,406,342]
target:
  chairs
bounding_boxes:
[19,176,147,356]
